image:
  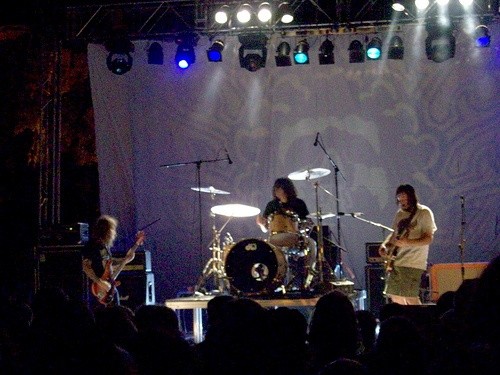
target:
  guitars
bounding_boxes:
[91,230,146,304]
[384,220,418,273]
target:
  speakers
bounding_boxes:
[429,262,489,301]
[32,245,156,312]
[311,225,342,279]
[364,265,427,317]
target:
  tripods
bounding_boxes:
[192,194,231,295]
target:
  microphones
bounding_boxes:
[314,133,320,146]
[225,149,233,165]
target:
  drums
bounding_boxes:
[282,234,311,256]
[267,207,301,246]
[221,241,236,271]
[225,238,286,296]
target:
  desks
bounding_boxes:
[165,290,370,343]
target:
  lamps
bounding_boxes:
[472,19,492,48]
[317,35,336,66]
[174,34,199,72]
[349,35,365,63]
[391,0,407,12]
[256,1,273,27]
[237,32,269,71]
[205,34,225,64]
[293,36,312,65]
[425,20,457,63]
[103,39,136,76]
[278,1,295,25]
[238,3,254,26]
[214,3,233,28]
[386,31,404,60]
[365,31,384,61]
[274,38,293,67]
[144,39,164,67]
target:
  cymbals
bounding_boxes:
[305,212,335,219]
[288,167,331,180]
[191,186,231,195]
[210,204,261,217]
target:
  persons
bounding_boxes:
[379,184,438,305]
[83,215,135,305]
[0,255,500,375]
[256,178,312,280]
